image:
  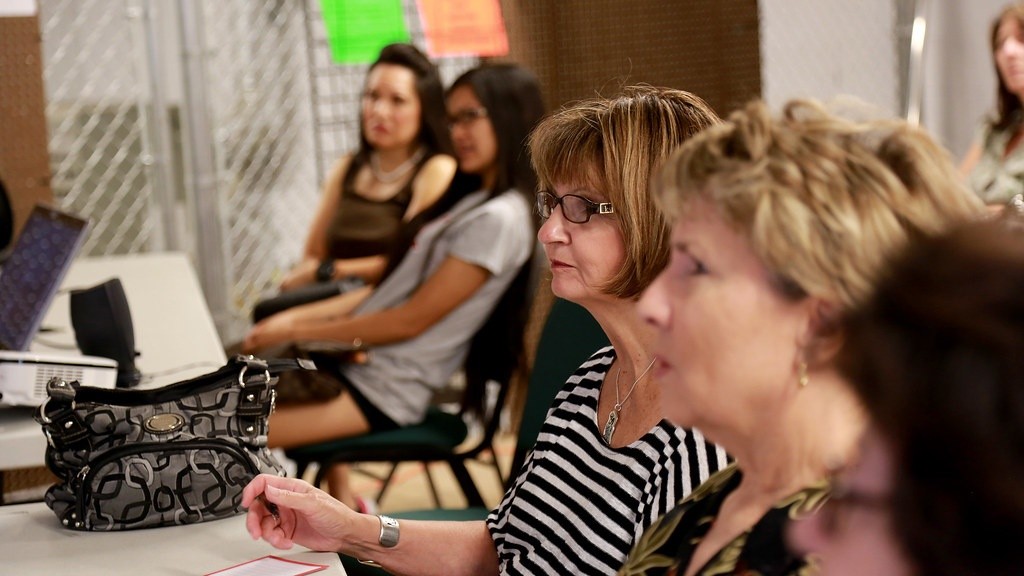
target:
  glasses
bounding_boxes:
[535,190,617,224]
[443,106,489,128]
[819,458,891,531]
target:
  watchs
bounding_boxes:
[316,257,337,283]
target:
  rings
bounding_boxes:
[272,513,281,529]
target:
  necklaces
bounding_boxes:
[602,356,658,447]
[368,147,425,179]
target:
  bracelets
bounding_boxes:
[357,515,400,569]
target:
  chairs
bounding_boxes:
[289,295,608,576]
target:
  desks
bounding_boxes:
[0,251,347,576]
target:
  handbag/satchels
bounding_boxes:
[37,351,290,532]
[271,345,341,404]
[250,283,341,324]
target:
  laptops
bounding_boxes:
[0,205,90,352]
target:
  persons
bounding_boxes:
[958,4,1024,208]
[253,42,460,326]
[618,95,987,576]
[243,63,547,517]
[782,215,1024,576]
[241,83,739,576]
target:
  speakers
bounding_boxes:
[71,279,139,390]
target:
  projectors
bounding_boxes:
[0,350,119,409]
